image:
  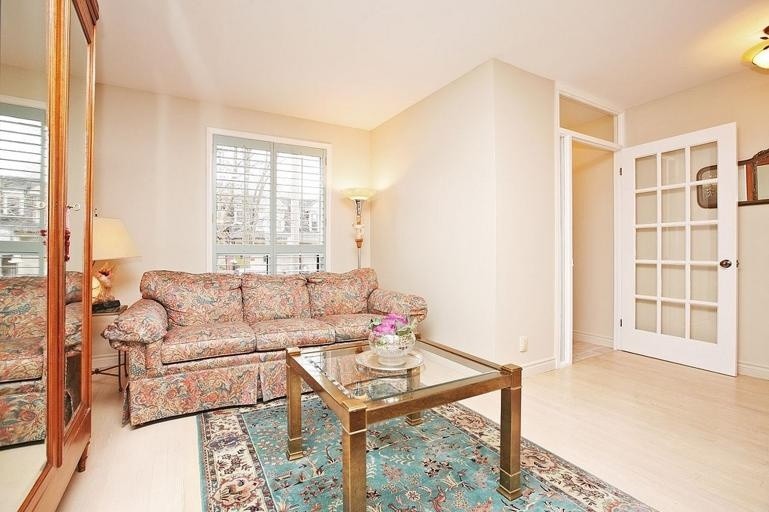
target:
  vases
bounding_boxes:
[365,311,418,351]
[369,332,416,365]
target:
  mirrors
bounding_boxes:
[62,1,89,438]
[0,2,52,508]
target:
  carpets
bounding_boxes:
[195,375,662,510]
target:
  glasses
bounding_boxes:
[742,26,769,72]
[92,219,139,309]
[341,187,373,269]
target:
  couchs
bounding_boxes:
[101,269,428,428]
[2,276,47,445]
[65,271,83,428]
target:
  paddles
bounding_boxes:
[195,375,662,510]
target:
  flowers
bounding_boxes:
[365,311,418,351]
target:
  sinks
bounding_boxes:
[101,269,428,428]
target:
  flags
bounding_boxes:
[369,332,416,365]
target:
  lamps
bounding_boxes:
[92,219,139,309]
[742,26,769,72]
[341,187,373,269]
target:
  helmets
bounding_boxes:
[354,348,425,372]
